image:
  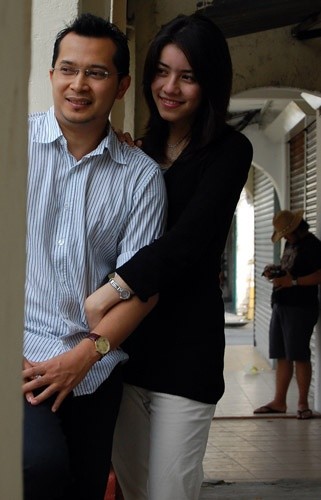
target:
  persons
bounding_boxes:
[253,209,321,419]
[21,12,168,500]
[85,13,255,500]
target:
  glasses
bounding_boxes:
[52,65,118,81]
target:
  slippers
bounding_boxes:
[253,405,286,414]
[297,409,314,420]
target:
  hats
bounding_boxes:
[270,210,304,243]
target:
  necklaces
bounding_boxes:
[167,132,189,150]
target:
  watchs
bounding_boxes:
[83,333,111,361]
[292,276,298,286]
[108,278,131,300]
